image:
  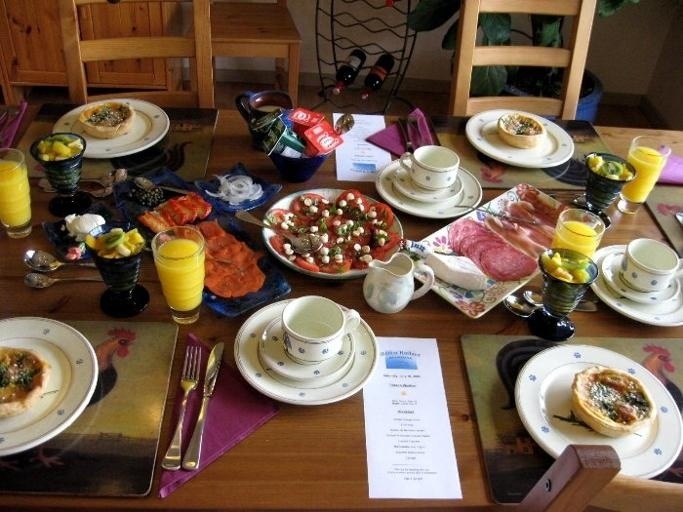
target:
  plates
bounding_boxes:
[52,97,170,158]
[601,251,680,302]
[43,204,131,259]
[407,182,600,320]
[261,188,404,279]
[260,315,356,379]
[114,166,292,317]
[393,163,463,202]
[513,344,682,485]
[195,161,280,214]
[376,158,482,217]
[587,244,683,326]
[233,297,379,405]
[464,108,573,168]
[0,316,98,462]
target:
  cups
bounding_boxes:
[616,136,672,213]
[621,237,683,295]
[152,226,206,326]
[85,224,150,317]
[553,208,606,259]
[586,153,638,210]
[537,248,599,315]
[0,147,33,238]
[282,295,359,366]
[401,145,460,190]
[236,91,291,150]
[30,133,91,215]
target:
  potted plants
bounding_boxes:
[407,1,604,123]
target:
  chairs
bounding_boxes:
[452,0,597,121]
[59,1,215,110]
[521,445,682,512]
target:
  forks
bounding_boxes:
[161,346,202,470]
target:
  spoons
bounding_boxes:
[523,290,598,307]
[24,273,103,289]
[234,209,323,254]
[399,117,415,169]
[25,248,97,272]
[501,295,596,324]
[135,177,198,197]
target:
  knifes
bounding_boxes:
[182,341,226,470]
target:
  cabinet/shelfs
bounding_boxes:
[1,1,183,106]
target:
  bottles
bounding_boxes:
[358,53,396,102]
[332,49,366,95]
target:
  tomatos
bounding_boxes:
[268,189,398,273]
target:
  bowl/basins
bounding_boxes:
[265,135,333,182]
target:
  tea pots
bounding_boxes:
[363,252,435,313]
